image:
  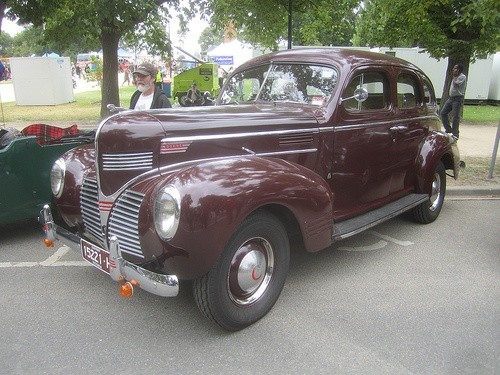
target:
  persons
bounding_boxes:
[129,62,172,110]
[216,63,225,89]
[76,58,187,90]
[184,83,205,107]
[440,64,467,144]
[2,64,9,82]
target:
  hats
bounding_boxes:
[132,62,159,77]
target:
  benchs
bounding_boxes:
[364,93,413,110]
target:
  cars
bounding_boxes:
[42,44,465,331]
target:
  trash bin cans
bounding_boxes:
[163,81,171,98]
